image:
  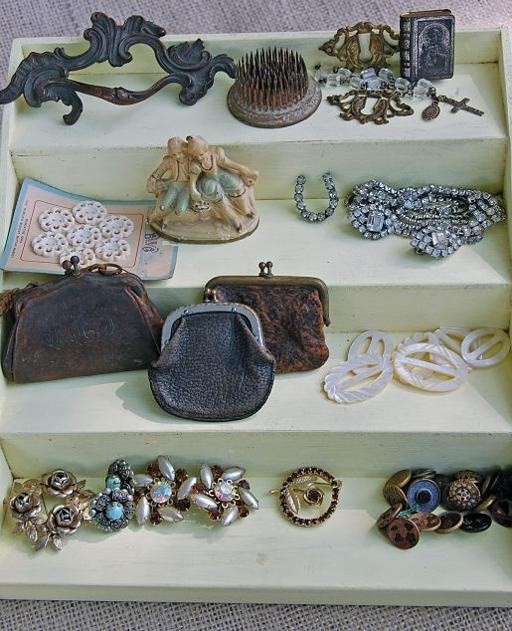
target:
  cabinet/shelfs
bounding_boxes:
[0,25,512,606]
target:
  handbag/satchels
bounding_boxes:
[147,289,276,421]
[205,260,330,372]
[2,256,161,383]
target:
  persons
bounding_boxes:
[143,134,196,229]
[184,135,260,237]
